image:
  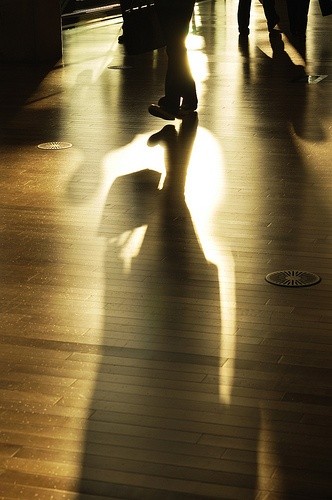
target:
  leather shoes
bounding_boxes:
[149,104,175,120]
[176,107,195,119]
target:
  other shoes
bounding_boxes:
[239,26,250,34]
[268,14,279,27]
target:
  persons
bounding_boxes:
[147,0,198,122]
[237,0,287,55]
